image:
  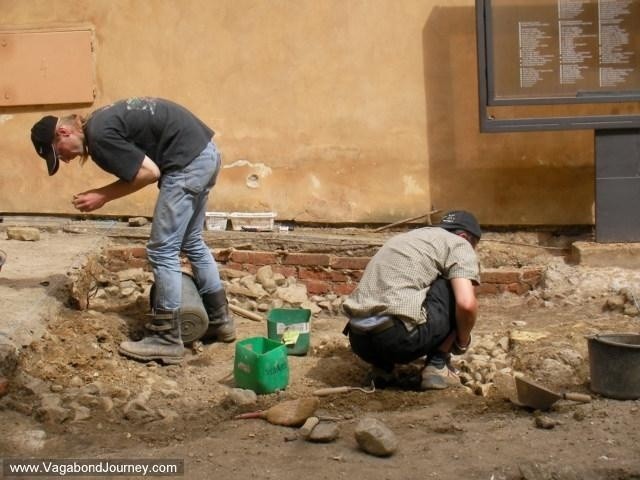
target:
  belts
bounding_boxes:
[342,318,398,335]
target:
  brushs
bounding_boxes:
[235,397,321,427]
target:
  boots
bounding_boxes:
[119,309,185,364]
[204,288,236,343]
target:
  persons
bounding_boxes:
[343,211,481,390]
[31,96,236,364]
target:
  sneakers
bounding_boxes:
[421,364,461,389]
[369,371,397,388]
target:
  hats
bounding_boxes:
[432,210,481,238]
[31,116,59,176]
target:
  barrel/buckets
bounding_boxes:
[267,308,311,355]
[149,271,209,345]
[234,337,288,393]
[583,332,640,400]
